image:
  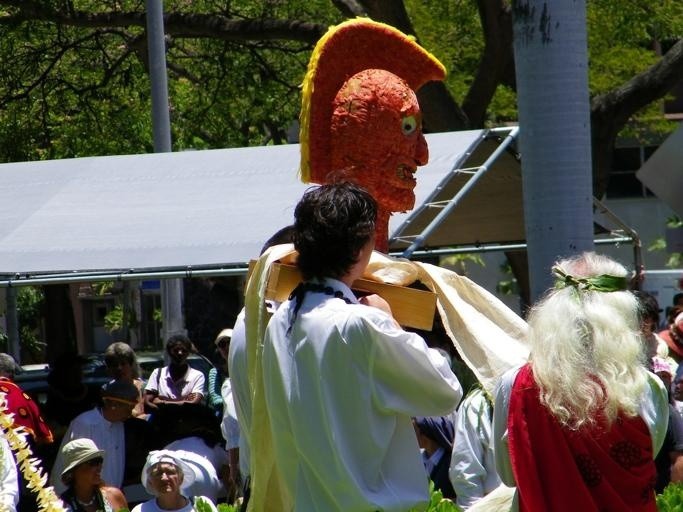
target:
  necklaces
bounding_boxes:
[283,282,351,337]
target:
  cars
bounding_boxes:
[14,355,112,406]
[131,347,222,396]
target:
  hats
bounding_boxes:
[141,450,195,495]
[60,437,104,476]
[214,328,233,345]
[649,356,671,377]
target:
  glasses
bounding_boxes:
[85,457,103,465]
[218,338,230,348]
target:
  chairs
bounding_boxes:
[10,354,243,500]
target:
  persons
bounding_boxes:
[493,252,672,511]
[299,20,446,254]
[0,293,681,510]
[259,180,465,512]
[229,224,319,510]
